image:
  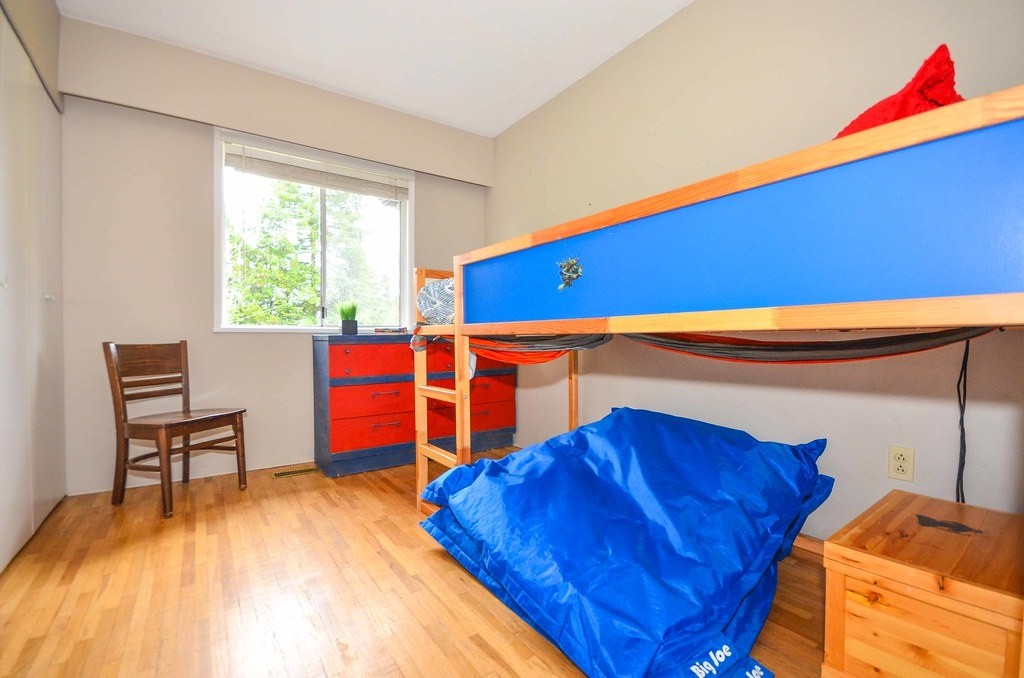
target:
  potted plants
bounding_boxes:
[339,302,357,335]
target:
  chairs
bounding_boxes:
[102,340,247,518]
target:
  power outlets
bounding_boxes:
[887,446,915,483]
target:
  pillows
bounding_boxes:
[834,44,966,140]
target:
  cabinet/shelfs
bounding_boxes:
[312,333,518,478]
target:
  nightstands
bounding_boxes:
[820,487,1024,677]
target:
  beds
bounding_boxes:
[413,82,1024,518]
